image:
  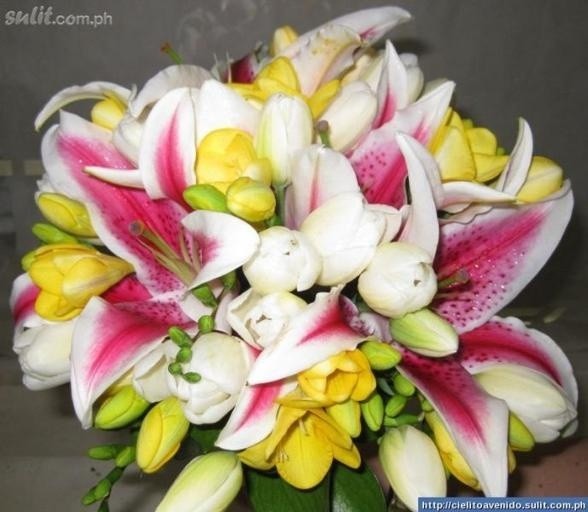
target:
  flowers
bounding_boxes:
[10,4,580,512]
